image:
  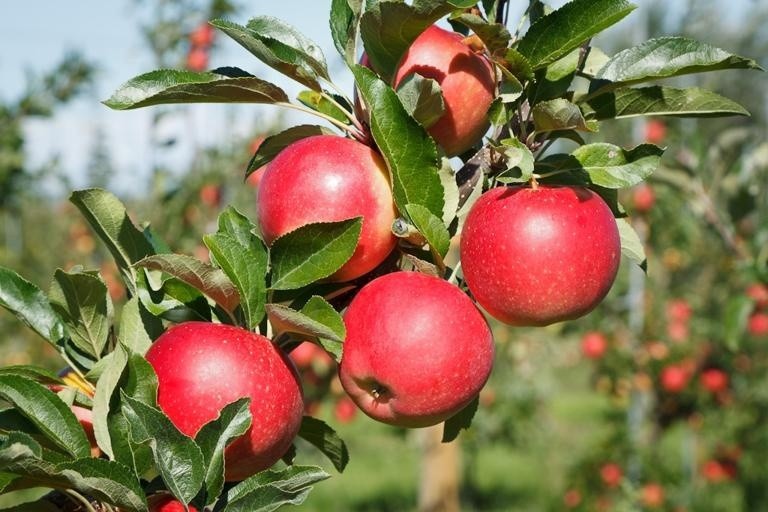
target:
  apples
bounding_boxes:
[0,1,767,511]
